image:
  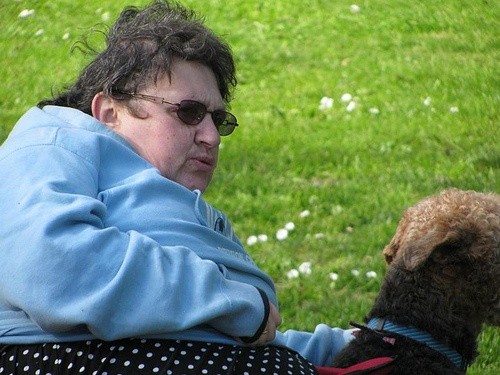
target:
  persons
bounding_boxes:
[0,0,364,375]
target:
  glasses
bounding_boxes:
[113,89,239,135]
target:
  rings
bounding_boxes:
[262,331,268,334]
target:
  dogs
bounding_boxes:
[327,186,500,375]
